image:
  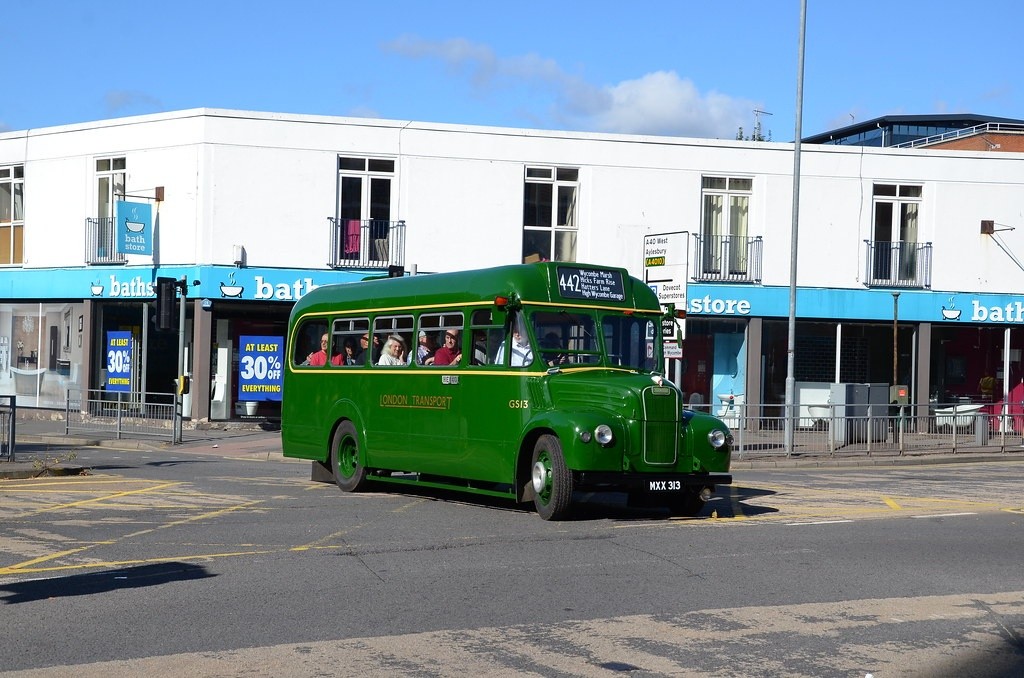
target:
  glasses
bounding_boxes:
[320,340,327,344]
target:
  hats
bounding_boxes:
[418,330,431,337]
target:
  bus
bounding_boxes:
[279,260,733,521]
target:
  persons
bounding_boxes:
[299,324,565,366]
[978,368,1000,401]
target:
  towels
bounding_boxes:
[343,218,360,253]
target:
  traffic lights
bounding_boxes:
[148,277,178,333]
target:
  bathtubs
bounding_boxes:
[928,404,984,428]
